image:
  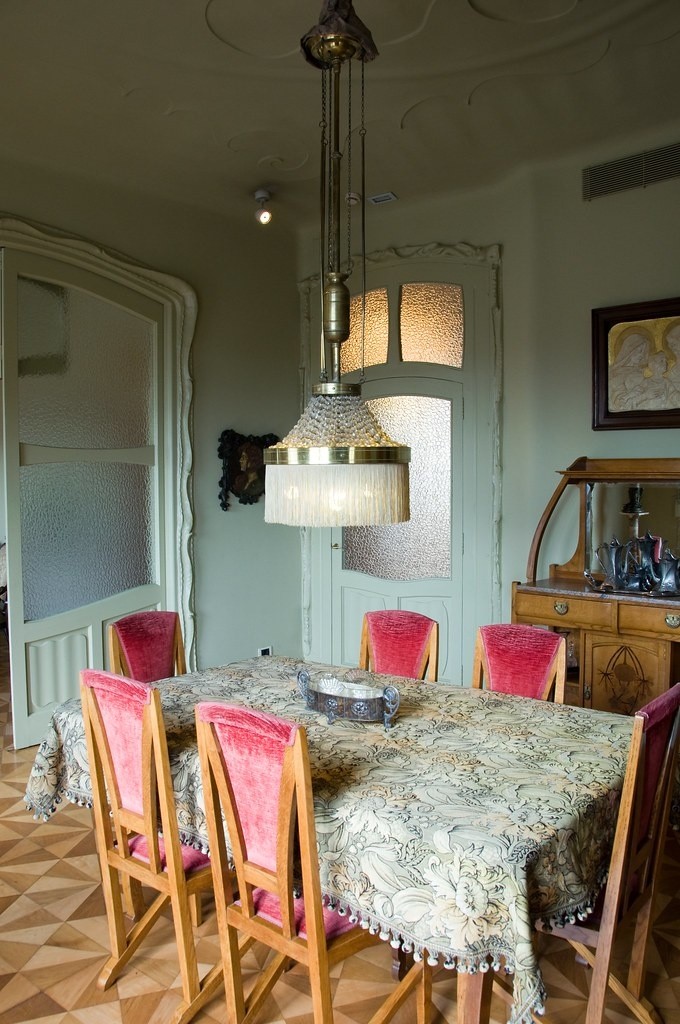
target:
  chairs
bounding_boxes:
[79,668,258,1023]
[464,683,679,1024]
[359,610,439,683]
[108,611,187,683]
[471,624,565,703]
[194,700,433,1024]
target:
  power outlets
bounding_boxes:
[258,646,272,657]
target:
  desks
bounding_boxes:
[22,658,634,1024]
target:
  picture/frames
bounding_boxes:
[217,429,281,512]
[591,297,680,431]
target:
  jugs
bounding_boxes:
[594,530,680,597]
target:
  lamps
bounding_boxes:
[264,0,411,528]
[253,190,272,226]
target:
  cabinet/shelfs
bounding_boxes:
[512,458,680,846]
